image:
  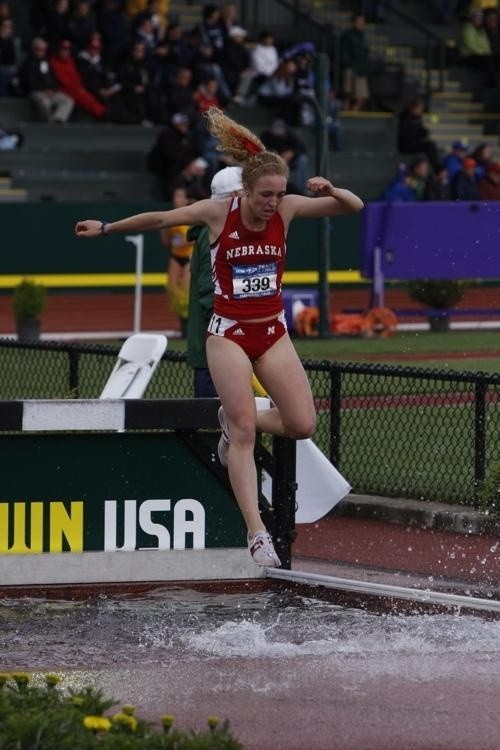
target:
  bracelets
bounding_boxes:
[101,220,110,237]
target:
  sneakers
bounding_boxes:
[246,530,282,567]
[217,404,231,468]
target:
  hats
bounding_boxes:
[210,165,245,194]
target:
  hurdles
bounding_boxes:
[1,400,297,596]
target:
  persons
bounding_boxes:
[340,16,368,110]
[147,114,231,199]
[398,99,438,164]
[159,186,201,338]
[74,102,366,567]
[461,7,500,87]
[183,165,242,397]
[259,119,310,195]
[2,2,339,123]
[296,54,344,150]
[382,141,500,200]
